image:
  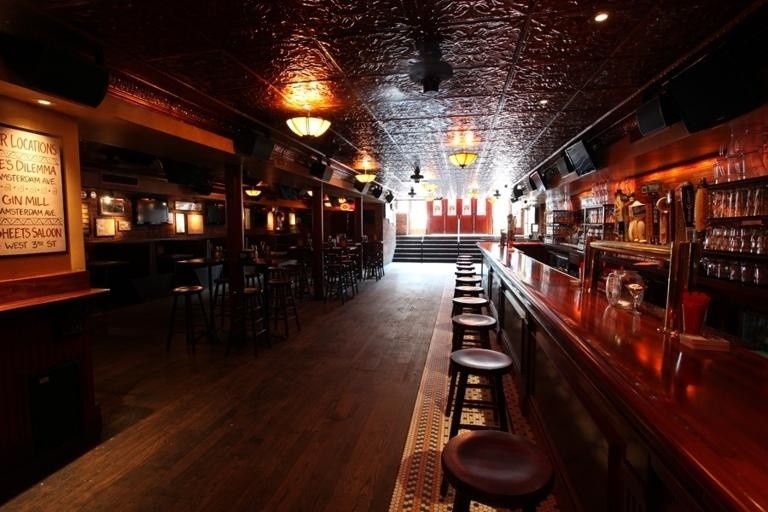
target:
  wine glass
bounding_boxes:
[260,242,265,251]
[625,283,648,316]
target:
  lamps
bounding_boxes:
[446,148,478,169]
[355,165,376,183]
[245,187,262,197]
[285,112,332,143]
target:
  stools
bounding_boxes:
[438,255,553,511]
[165,238,383,357]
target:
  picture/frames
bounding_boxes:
[0,123,69,258]
[96,195,127,217]
[79,200,91,235]
[175,211,185,234]
[186,213,206,236]
[95,218,116,238]
[118,220,131,232]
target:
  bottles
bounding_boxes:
[701,187,768,254]
[587,209,604,238]
[713,122,768,184]
[606,271,644,310]
[590,180,609,208]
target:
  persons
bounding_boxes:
[508,214,516,241]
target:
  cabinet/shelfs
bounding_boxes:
[542,204,617,245]
[693,176,767,288]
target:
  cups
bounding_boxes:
[682,302,709,337]
[212,246,222,260]
[656,240,691,338]
